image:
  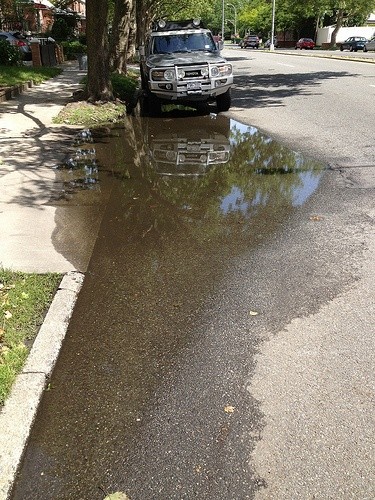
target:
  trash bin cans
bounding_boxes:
[40,44,57,67]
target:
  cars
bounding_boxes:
[0,32,31,60]
[340,37,368,52]
[240,34,260,49]
[18,30,55,46]
[295,38,315,50]
[264,36,279,48]
[362,32,375,52]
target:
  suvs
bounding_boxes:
[140,18,233,114]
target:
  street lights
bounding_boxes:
[226,3,237,38]
